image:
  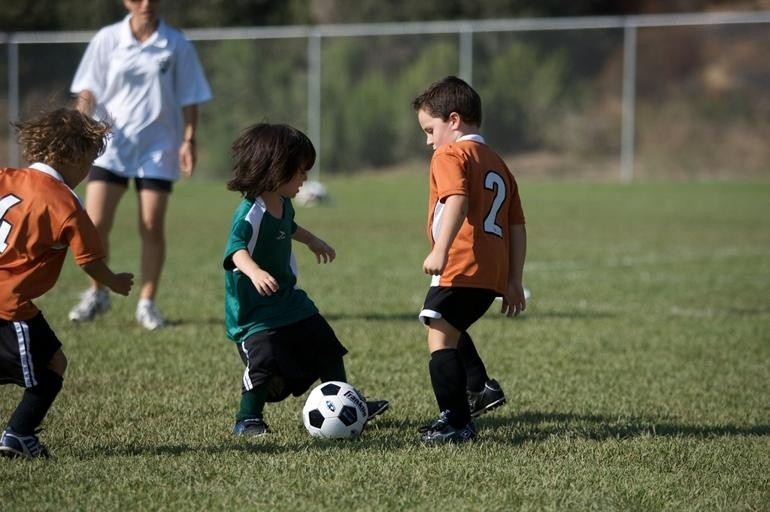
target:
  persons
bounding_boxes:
[414,74,527,440]
[0,107,135,461]
[223,122,390,440]
[67,3,212,330]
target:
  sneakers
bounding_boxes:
[467,378,506,418]
[233,418,267,434]
[367,400,390,420]
[136,298,163,331]
[0,426,50,460]
[69,286,112,323]
[423,410,477,442]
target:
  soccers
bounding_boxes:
[303,381,368,441]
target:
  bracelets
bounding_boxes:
[182,138,193,143]
